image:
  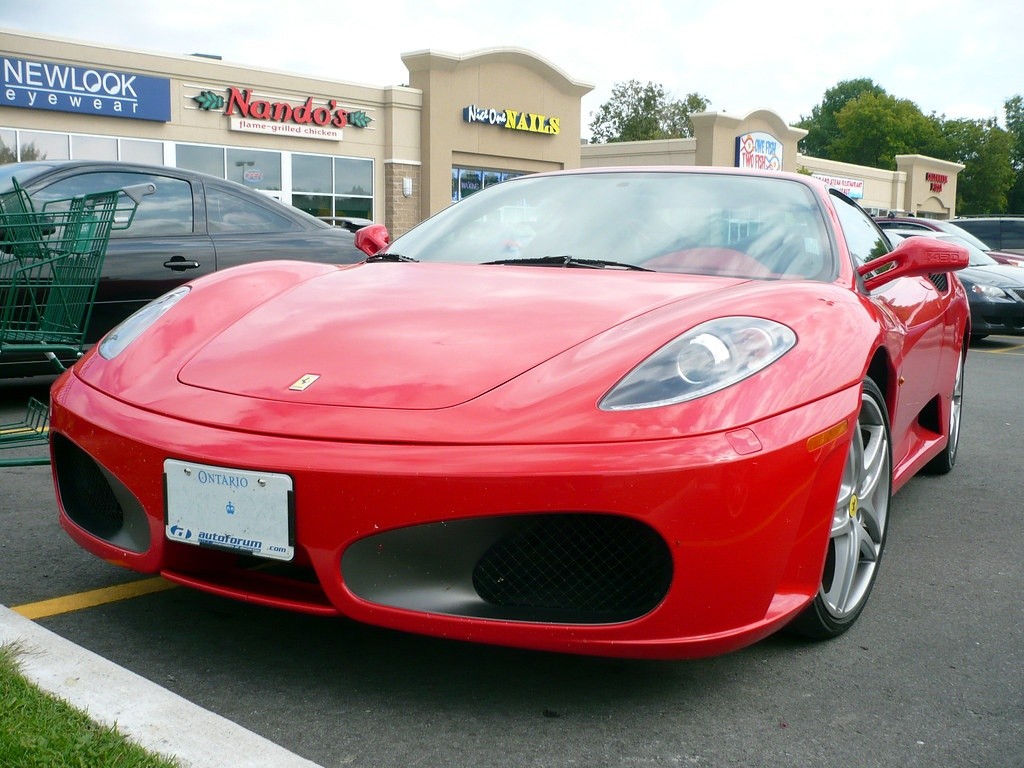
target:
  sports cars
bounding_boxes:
[48,166,971,660]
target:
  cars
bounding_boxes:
[1,159,370,381]
[881,228,1024,341]
[869,215,1023,268]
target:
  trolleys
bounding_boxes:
[0,173,156,466]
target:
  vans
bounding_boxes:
[944,218,1024,258]
